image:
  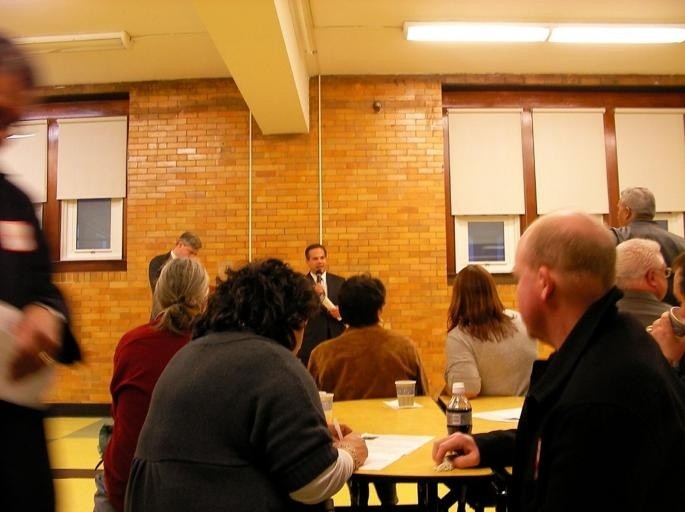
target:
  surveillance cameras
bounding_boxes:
[374,102,381,111]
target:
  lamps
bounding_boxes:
[0,31,134,60]
[403,21,685,44]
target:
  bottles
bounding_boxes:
[447,382,472,456]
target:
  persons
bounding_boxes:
[0,33,87,512]
[432,188,684,511]
[102,230,430,511]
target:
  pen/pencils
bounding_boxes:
[334,419,343,441]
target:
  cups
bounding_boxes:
[670,306,685,337]
[318,391,334,425]
[395,380,416,407]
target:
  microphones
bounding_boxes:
[316,269,322,284]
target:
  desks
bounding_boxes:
[323,395,494,512]
[436,395,527,512]
